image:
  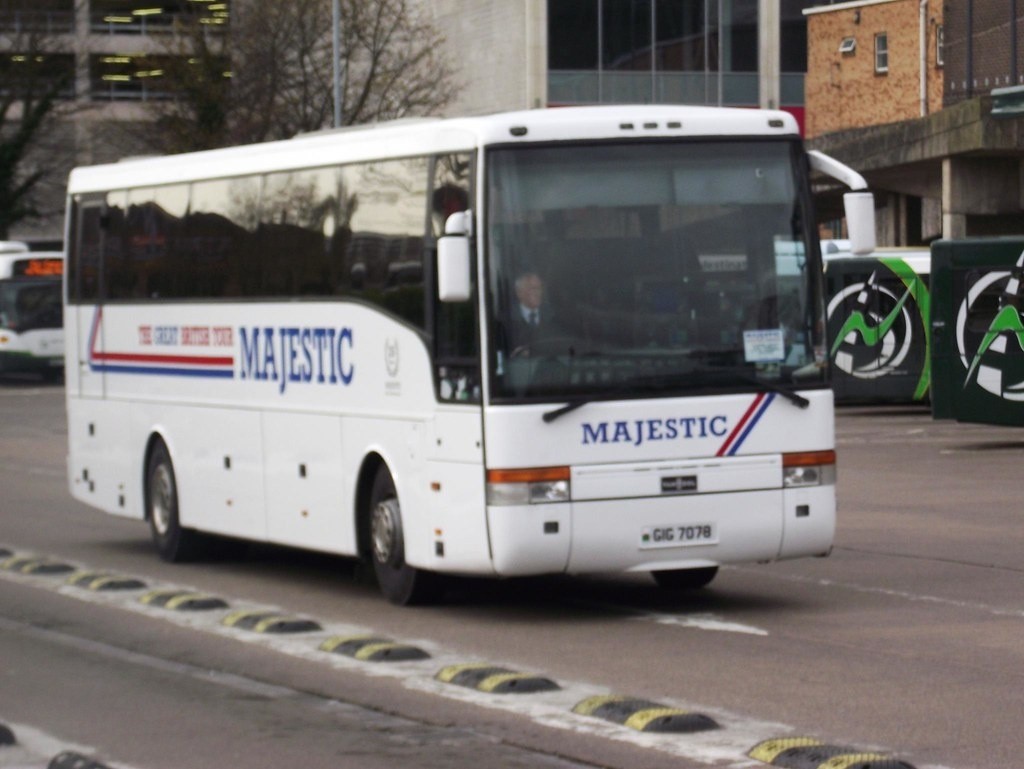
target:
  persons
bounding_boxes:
[504,273,582,358]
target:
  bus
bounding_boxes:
[63,105,876,605]
[0,241,65,371]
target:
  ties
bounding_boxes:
[529,311,536,325]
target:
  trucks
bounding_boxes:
[929,234,1024,428]
[695,239,931,404]
